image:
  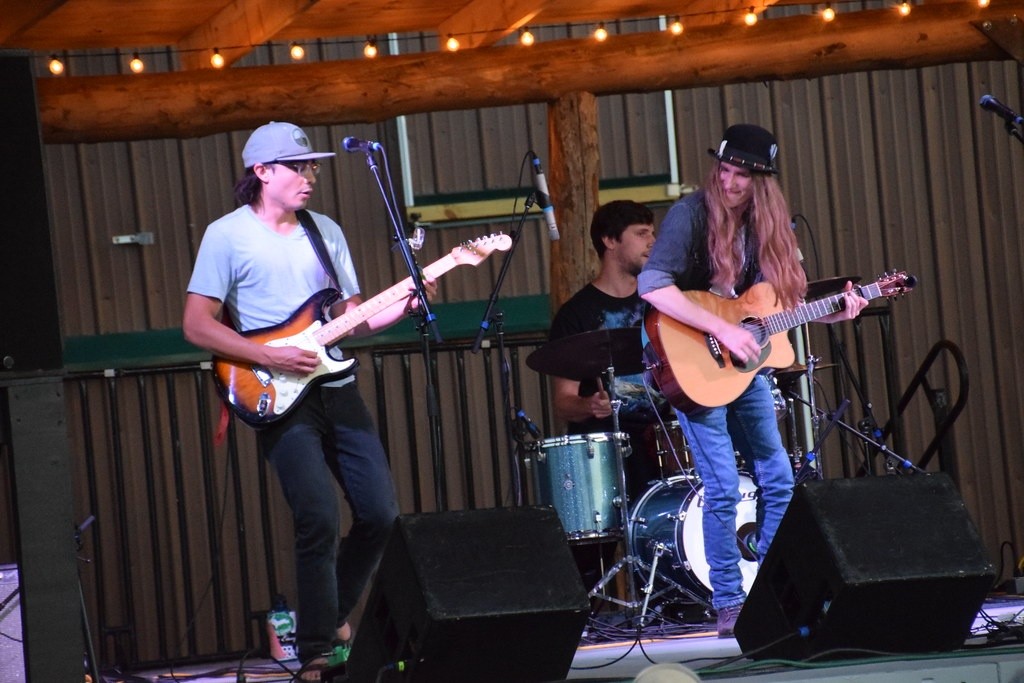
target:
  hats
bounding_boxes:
[241,121,336,168]
[707,124,779,174]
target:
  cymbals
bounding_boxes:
[525,325,647,382]
[775,361,838,376]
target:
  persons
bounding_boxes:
[549,199,679,638]
[637,125,869,638]
[183,121,437,683]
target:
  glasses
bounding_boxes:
[265,161,322,177]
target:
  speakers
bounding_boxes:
[0,563,29,683]
[733,473,997,666]
[346,504,592,683]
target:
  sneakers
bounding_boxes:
[716,604,743,639]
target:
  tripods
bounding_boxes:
[589,345,719,626]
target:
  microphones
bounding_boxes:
[532,153,560,241]
[980,94,1024,126]
[513,406,542,439]
[790,216,797,231]
[342,136,381,152]
[743,532,758,553]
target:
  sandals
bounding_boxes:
[298,657,330,682]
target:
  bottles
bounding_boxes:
[266,594,297,661]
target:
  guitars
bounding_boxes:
[642,266,919,418]
[209,228,514,432]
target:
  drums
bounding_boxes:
[654,373,789,432]
[624,475,761,602]
[519,432,628,546]
